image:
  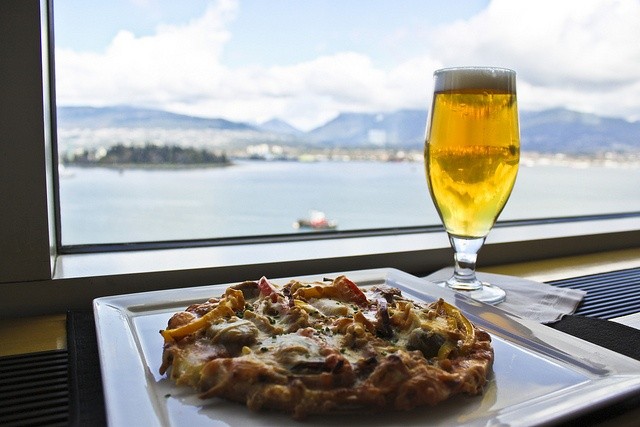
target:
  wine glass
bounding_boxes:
[423,65,522,306]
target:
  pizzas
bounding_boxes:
[157,276,495,417]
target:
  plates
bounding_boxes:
[91,266,640,427]
